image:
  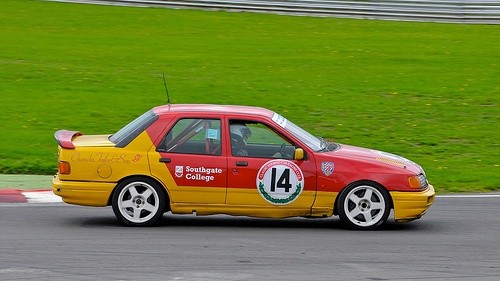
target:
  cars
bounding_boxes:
[53,104,436,231]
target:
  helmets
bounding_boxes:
[230,124,252,149]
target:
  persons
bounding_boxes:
[230,123,283,158]
